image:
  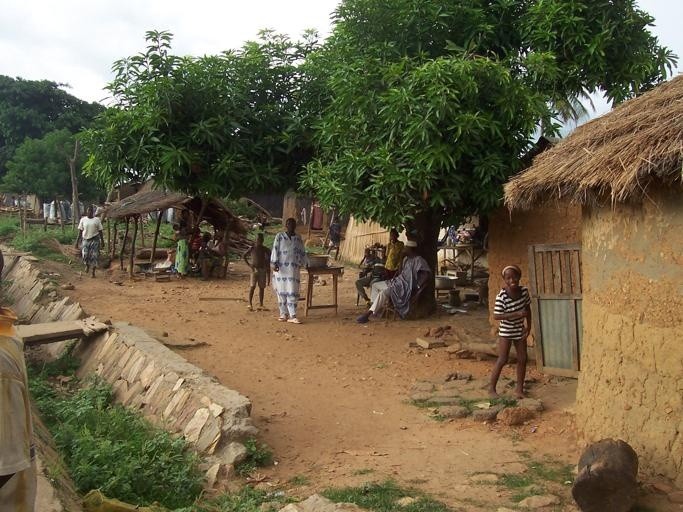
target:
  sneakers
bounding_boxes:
[356,316,368,323]
[278,315,287,322]
[287,318,302,324]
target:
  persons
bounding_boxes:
[355,241,430,323]
[74,205,104,278]
[355,229,404,308]
[166,209,226,281]
[270,218,305,323]
[243,233,271,311]
[487,264,531,399]
[0,247,37,512]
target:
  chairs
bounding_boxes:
[386,269,427,321]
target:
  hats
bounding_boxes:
[406,241,417,246]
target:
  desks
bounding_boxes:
[437,239,487,281]
[304,262,344,317]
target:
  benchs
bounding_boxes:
[357,271,367,306]
